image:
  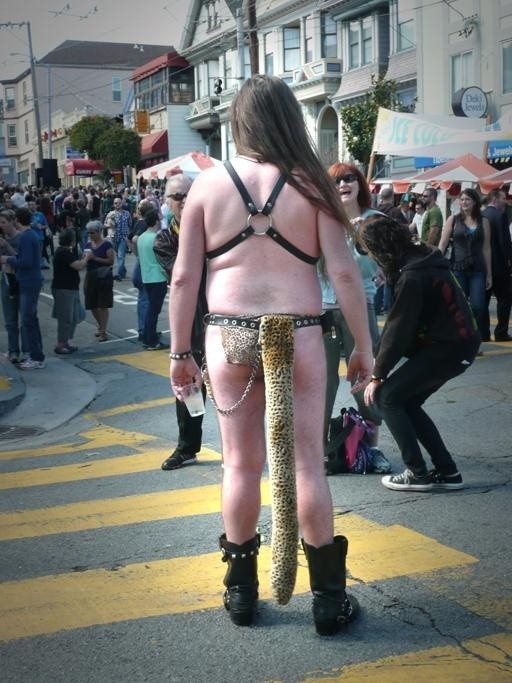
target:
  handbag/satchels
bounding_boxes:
[326,408,368,475]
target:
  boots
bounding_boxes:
[217,534,261,627]
[302,535,360,636]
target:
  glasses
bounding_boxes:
[167,193,185,202]
[336,174,356,184]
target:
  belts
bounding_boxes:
[206,313,319,328]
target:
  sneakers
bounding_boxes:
[162,447,196,468]
[139,330,169,350]
[55,345,78,355]
[382,472,431,491]
[9,352,45,370]
[369,448,390,473]
[430,470,464,490]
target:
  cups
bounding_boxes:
[84,248,91,255]
[179,380,206,418]
